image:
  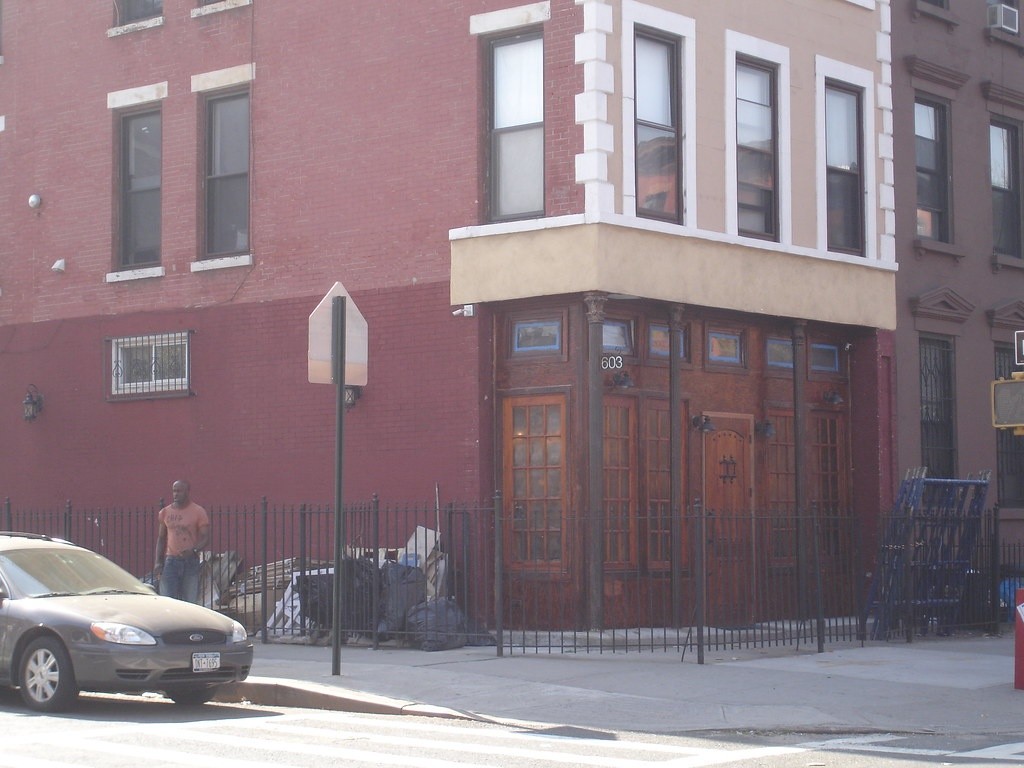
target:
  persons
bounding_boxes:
[154,481,210,605]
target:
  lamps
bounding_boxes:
[452,304,474,317]
[50,258,66,272]
[824,390,843,404]
[344,385,362,411]
[692,414,716,433]
[755,418,775,439]
[612,371,635,390]
[21,383,43,421]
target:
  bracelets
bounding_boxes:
[193,547,199,553]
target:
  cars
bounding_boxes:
[0,531,252,713]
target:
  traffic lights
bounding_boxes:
[992,375,1024,432]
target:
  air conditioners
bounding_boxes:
[986,2,1019,35]
